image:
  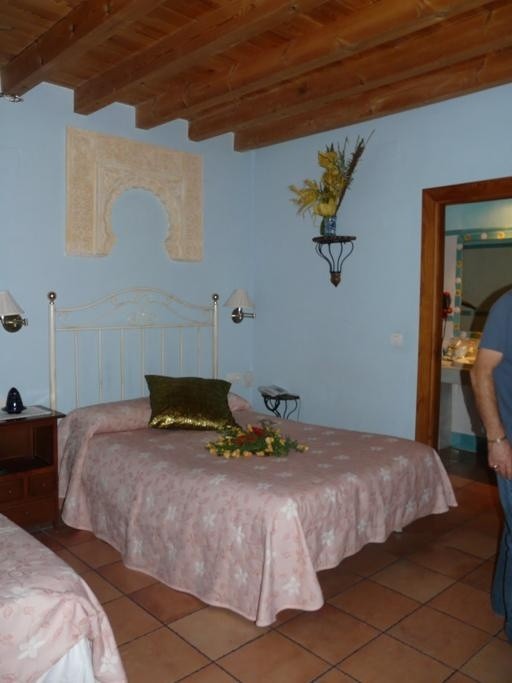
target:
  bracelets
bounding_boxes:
[484,434,509,444]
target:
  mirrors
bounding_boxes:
[451,228,512,338]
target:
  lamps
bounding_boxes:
[0,291,29,334]
[223,289,256,323]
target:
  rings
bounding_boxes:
[493,464,500,471]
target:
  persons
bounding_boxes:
[469,289,512,645]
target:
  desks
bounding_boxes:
[262,393,301,418]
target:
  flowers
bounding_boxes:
[288,128,375,225]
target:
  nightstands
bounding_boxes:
[0,405,66,530]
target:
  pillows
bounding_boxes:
[144,374,243,432]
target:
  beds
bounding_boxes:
[48,286,457,626]
[0,511,131,683]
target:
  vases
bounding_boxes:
[323,216,337,235]
